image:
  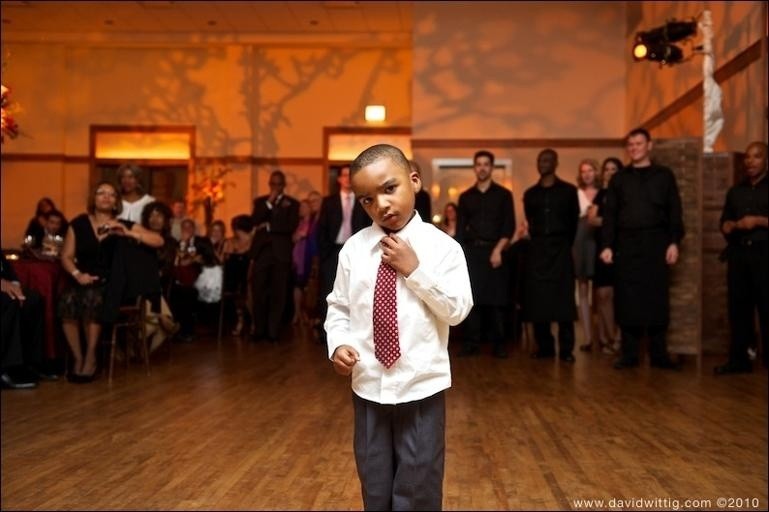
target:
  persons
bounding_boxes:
[411,129,683,371]
[24,163,372,385]
[710,141,769,375]
[0,245,57,388]
[323,145,475,511]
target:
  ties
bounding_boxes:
[370,231,403,371]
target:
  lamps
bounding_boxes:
[631,32,684,68]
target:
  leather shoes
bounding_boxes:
[610,356,642,371]
[456,348,481,357]
[559,353,575,363]
[494,348,509,359]
[66,361,104,385]
[580,335,623,355]
[713,360,754,375]
[529,350,556,360]
[1,370,39,392]
[19,355,63,380]
[649,354,682,371]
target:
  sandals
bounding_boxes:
[230,316,257,337]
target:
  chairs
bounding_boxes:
[0,221,259,392]
